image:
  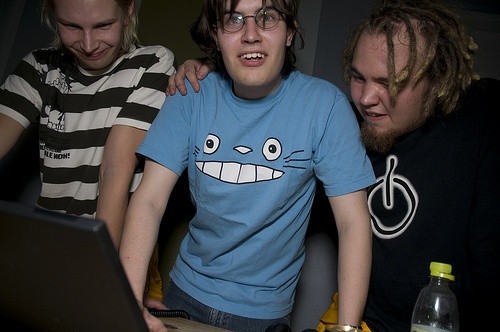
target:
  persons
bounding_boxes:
[0,0,176,256]
[163,0,500,332]
[143,243,171,310]
[118,1,377,332]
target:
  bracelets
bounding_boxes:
[324,322,363,332]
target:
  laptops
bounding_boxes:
[0,201,149,332]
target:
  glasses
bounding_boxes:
[213,7,287,32]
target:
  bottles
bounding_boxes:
[411,261,460,332]
